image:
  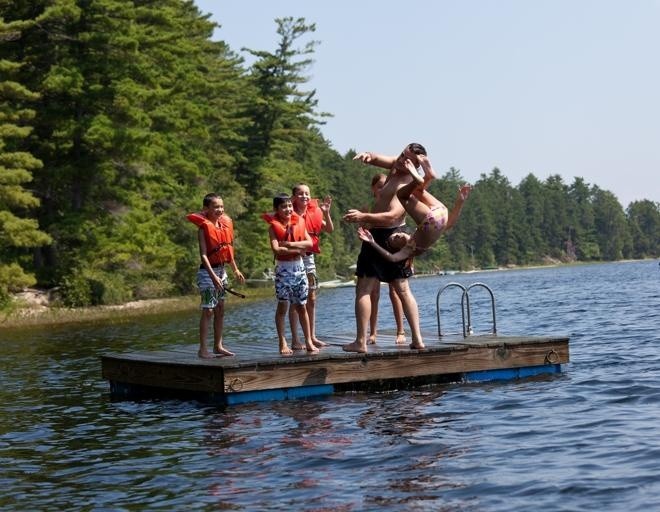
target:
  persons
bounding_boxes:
[288,183,335,350]
[197,193,245,359]
[269,193,320,354]
[342,143,474,353]
